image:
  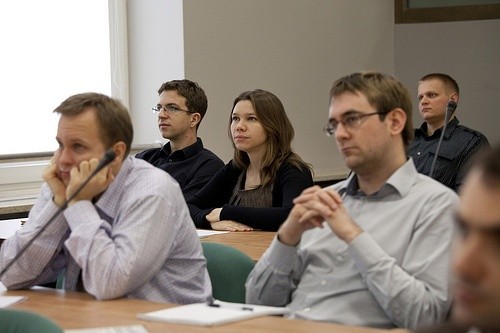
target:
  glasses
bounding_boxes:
[152,105,195,114]
[324,112,389,136]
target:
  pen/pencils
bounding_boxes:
[209,304,253,311]
[338,188,347,196]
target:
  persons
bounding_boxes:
[406,73,492,194]
[134,79,225,201]
[245,71,460,333]
[186,89,314,232]
[450,144,500,333]
[0,93,213,305]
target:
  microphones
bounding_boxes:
[429,101,458,177]
[0,150,117,279]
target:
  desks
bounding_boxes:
[0,281,425,333]
[196,228,278,262]
